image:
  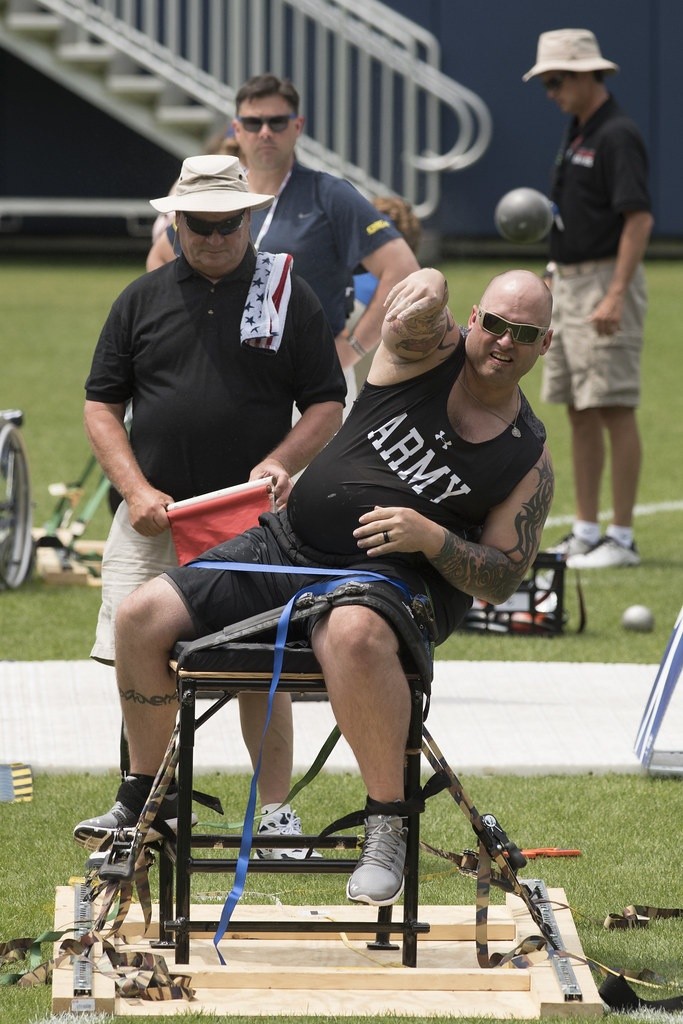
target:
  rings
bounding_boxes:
[383,530,389,543]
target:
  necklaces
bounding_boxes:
[456,377,520,438]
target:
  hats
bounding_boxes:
[521,28,621,82]
[150,155,275,214]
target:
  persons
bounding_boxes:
[152,122,248,239]
[148,75,419,483]
[521,29,655,568]
[78,154,347,859]
[71,269,555,906]
[373,199,418,256]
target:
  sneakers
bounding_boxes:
[346,800,409,907]
[72,770,198,852]
[566,536,641,568]
[86,849,156,871]
[544,533,595,559]
[252,811,323,859]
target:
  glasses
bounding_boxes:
[478,304,549,346]
[183,210,247,237]
[544,73,567,93]
[235,114,297,133]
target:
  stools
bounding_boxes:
[167,617,432,968]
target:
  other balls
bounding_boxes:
[621,604,653,631]
[495,188,554,243]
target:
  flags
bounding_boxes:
[165,477,275,567]
